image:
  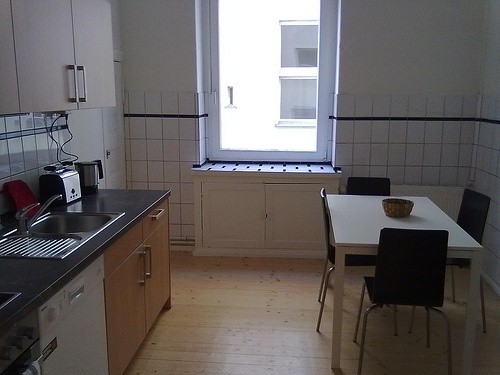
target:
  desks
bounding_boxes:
[326,194,483,375]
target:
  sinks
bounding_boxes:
[0,292,22,310]
[29,210,126,235]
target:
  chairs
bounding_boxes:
[316,189,398,338]
[347,177,392,196]
[445,189,491,335]
[354,228,453,375]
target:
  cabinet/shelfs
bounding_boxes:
[0,0,117,114]
[193,175,341,258]
[104,197,172,375]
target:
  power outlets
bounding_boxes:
[58,110,73,114]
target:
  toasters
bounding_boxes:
[38,161,82,206]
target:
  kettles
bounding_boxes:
[73,159,104,195]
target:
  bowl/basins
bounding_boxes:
[381,198,414,218]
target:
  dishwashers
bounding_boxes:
[37,254,108,375]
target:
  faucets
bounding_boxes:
[15,193,63,234]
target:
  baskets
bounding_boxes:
[382,198,414,217]
